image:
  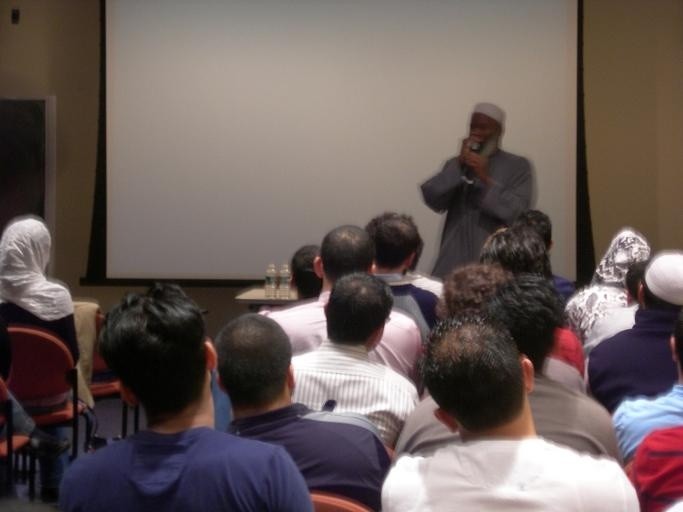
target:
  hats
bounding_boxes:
[645,254,683,306]
[474,103,503,123]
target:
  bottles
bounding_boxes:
[264,263,291,300]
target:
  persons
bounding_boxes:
[62,281,314,509]
[0,219,94,512]
[417,101,533,285]
[211,211,639,511]
[566,228,683,511]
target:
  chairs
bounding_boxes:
[68,296,142,446]
[0,326,99,483]
[305,483,376,512]
[0,371,36,512]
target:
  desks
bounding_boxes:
[232,280,298,320]
